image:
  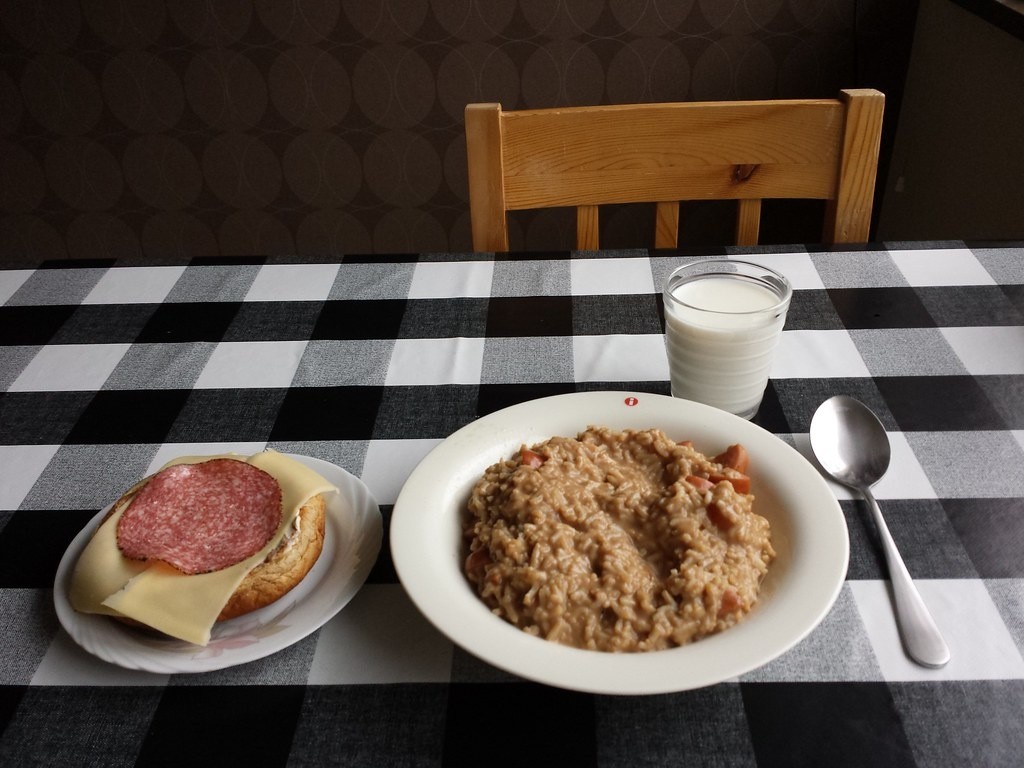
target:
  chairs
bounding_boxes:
[464,89,885,252]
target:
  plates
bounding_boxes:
[54,454,383,673]
[390,391,851,695]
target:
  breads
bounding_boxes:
[96,469,326,629]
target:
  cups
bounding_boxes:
[663,260,792,421]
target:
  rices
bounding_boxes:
[464,423,776,651]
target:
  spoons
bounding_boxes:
[810,394,949,668]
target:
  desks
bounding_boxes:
[0,239,1024,768]
